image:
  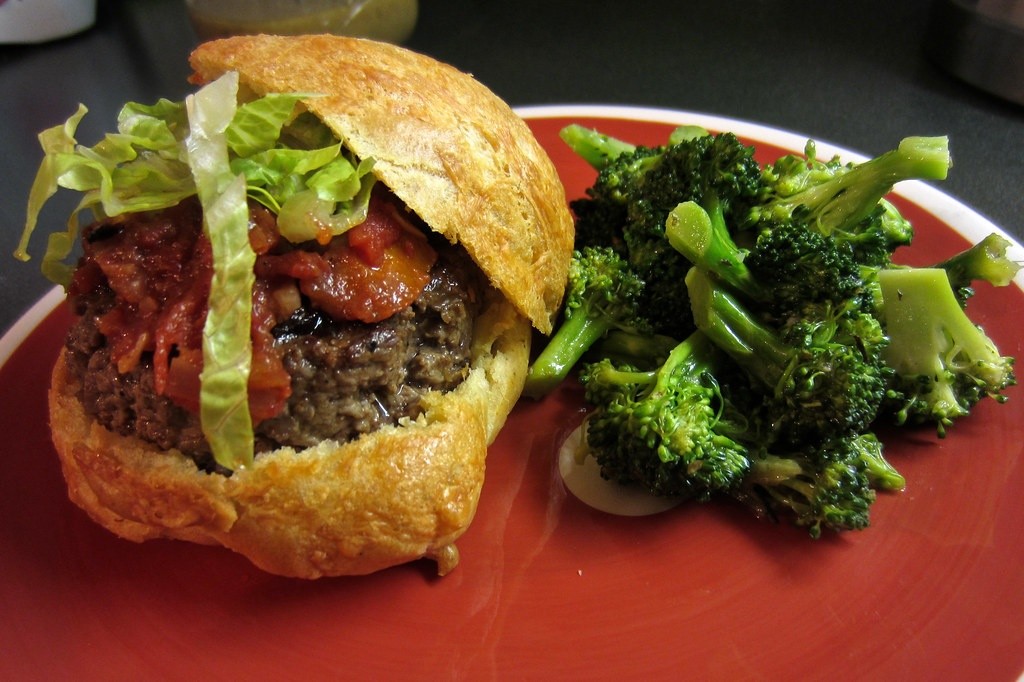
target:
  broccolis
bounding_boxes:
[524,125,1020,537]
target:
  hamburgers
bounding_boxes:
[15,36,575,578]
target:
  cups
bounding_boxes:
[0,0,97,43]
[185,0,418,47]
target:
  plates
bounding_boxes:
[0,102,1024,682]
